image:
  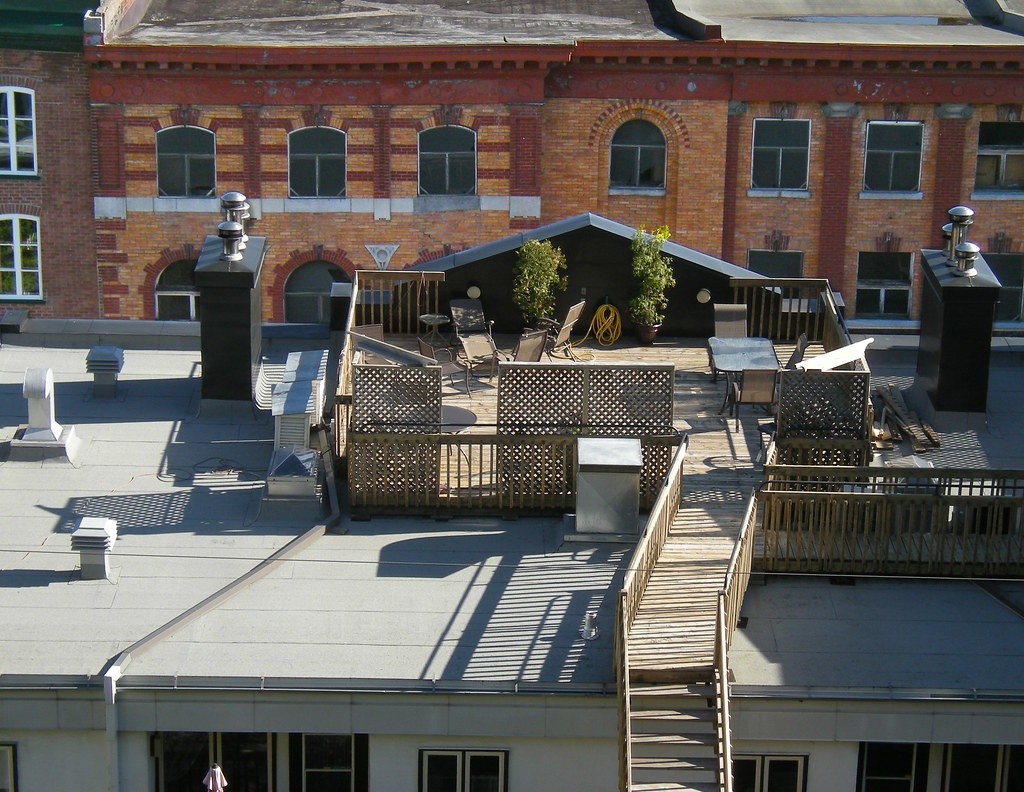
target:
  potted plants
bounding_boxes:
[627,225,676,344]
[512,239,567,337]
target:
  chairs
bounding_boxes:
[490,329,547,385]
[525,301,585,364]
[718,333,809,417]
[707,304,748,384]
[730,369,777,433]
[448,299,496,378]
[416,337,473,399]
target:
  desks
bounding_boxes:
[418,313,451,347]
[708,336,779,416]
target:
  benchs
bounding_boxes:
[755,418,778,463]
